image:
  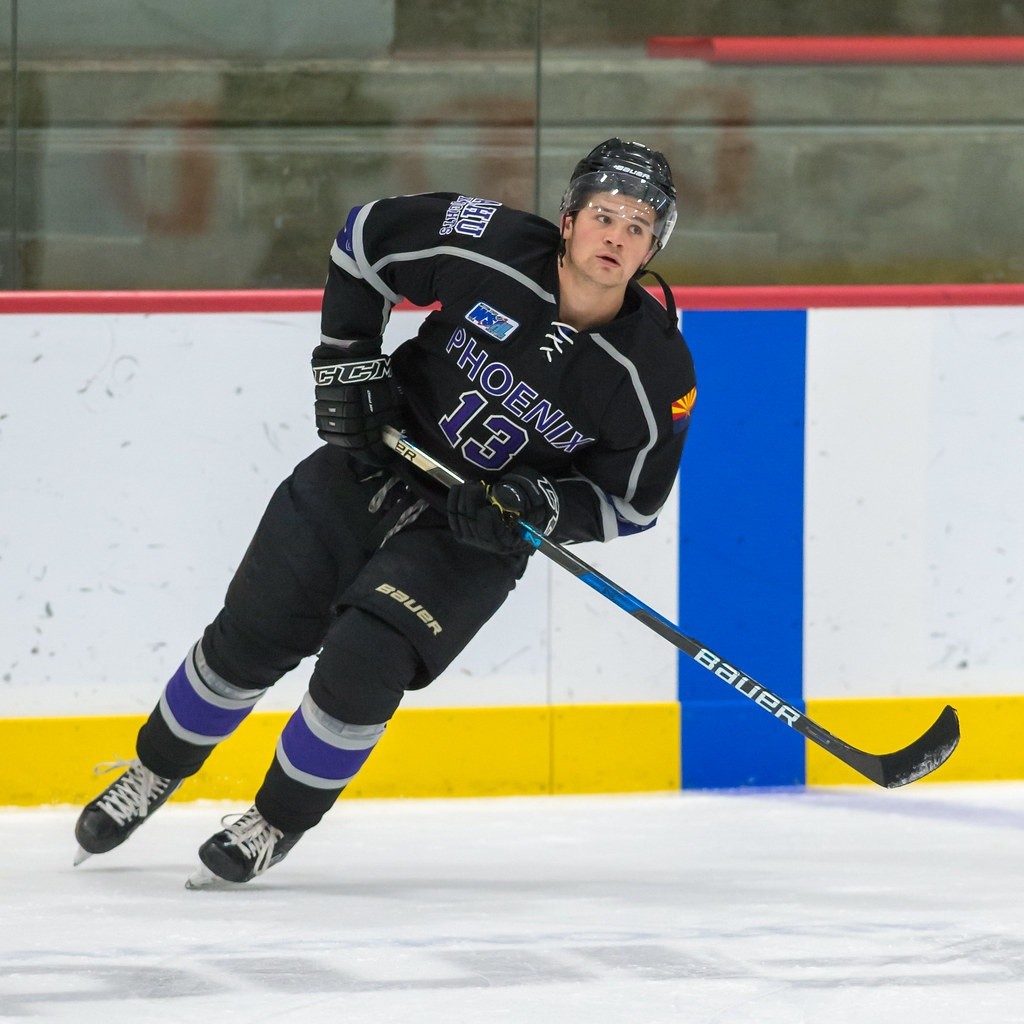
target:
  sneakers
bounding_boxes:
[66,762,179,872]
[190,806,301,893]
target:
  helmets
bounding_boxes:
[567,136,677,223]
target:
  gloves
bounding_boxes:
[445,471,551,557]
[310,340,397,452]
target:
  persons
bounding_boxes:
[72,136,696,891]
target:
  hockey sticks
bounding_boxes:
[373,413,962,791]
